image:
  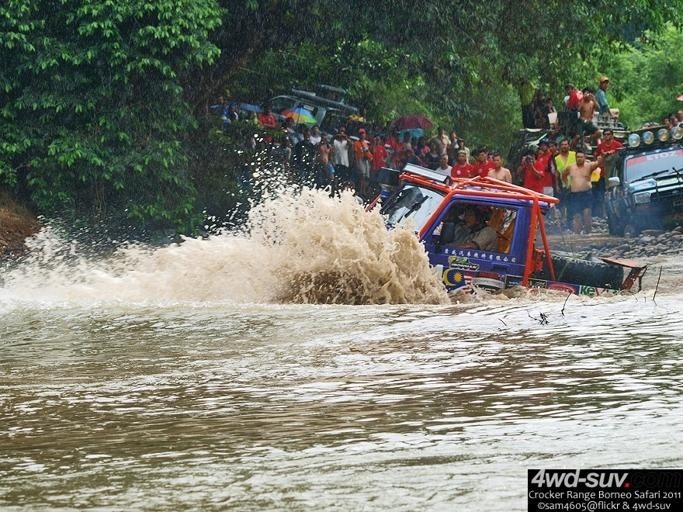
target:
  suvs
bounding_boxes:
[510,120,631,188]
[604,125,683,234]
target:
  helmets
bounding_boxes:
[465,203,493,221]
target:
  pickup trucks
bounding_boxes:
[229,89,393,166]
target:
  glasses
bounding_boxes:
[603,133,611,136]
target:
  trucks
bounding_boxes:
[381,161,648,296]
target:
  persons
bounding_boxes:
[211,74,683,252]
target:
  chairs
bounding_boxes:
[488,209,515,253]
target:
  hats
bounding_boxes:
[392,132,399,136]
[600,76,610,84]
[358,128,365,135]
